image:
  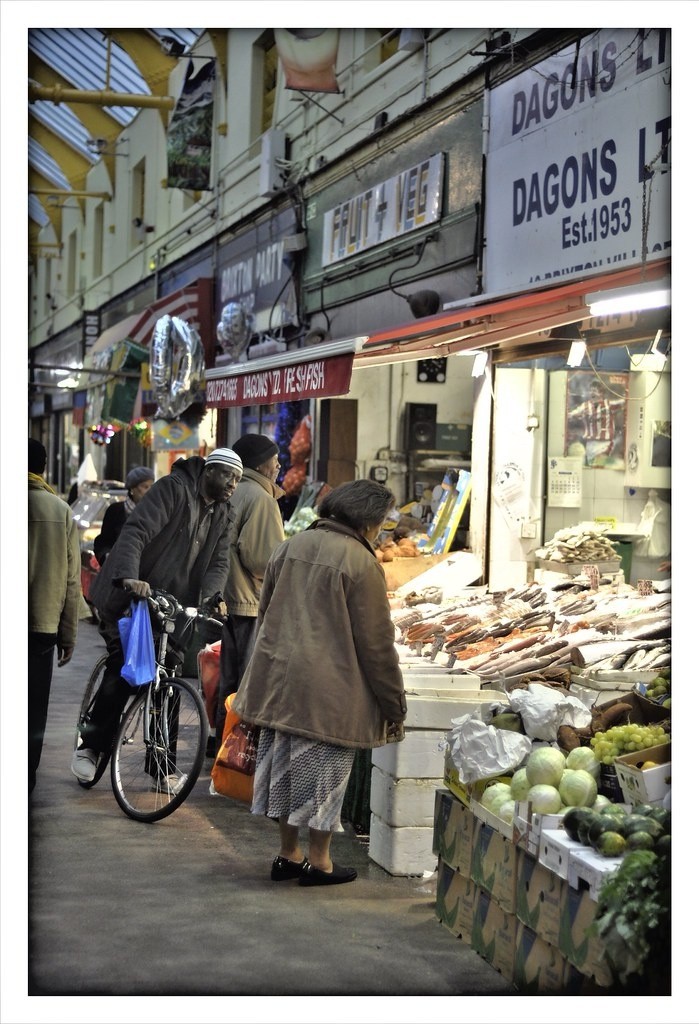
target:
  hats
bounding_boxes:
[204,448,244,474]
[125,467,155,489]
[232,433,280,468]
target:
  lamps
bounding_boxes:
[584,277,671,317]
[386,228,444,315]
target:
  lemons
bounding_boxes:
[645,677,670,710]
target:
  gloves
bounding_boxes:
[386,721,405,743]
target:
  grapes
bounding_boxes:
[590,723,670,765]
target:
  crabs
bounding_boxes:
[509,668,571,692]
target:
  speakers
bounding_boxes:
[404,402,436,450]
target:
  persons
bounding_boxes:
[70,447,245,796]
[93,466,154,568]
[231,479,408,887]
[215,434,287,762]
[28,437,81,797]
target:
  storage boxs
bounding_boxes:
[539,557,622,575]
[368,641,672,996]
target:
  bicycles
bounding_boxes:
[73,585,229,823]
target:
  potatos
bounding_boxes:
[560,703,632,752]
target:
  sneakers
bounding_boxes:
[150,774,184,795]
[71,745,99,781]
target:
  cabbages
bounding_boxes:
[481,746,612,824]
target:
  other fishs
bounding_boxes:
[534,529,622,563]
[443,579,671,680]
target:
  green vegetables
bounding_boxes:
[584,851,667,976]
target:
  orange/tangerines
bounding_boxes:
[282,429,311,496]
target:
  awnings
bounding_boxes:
[201,257,671,409]
[74,286,201,392]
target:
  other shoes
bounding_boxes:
[271,856,310,881]
[298,862,358,885]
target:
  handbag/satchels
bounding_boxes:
[118,597,157,687]
[209,693,260,810]
